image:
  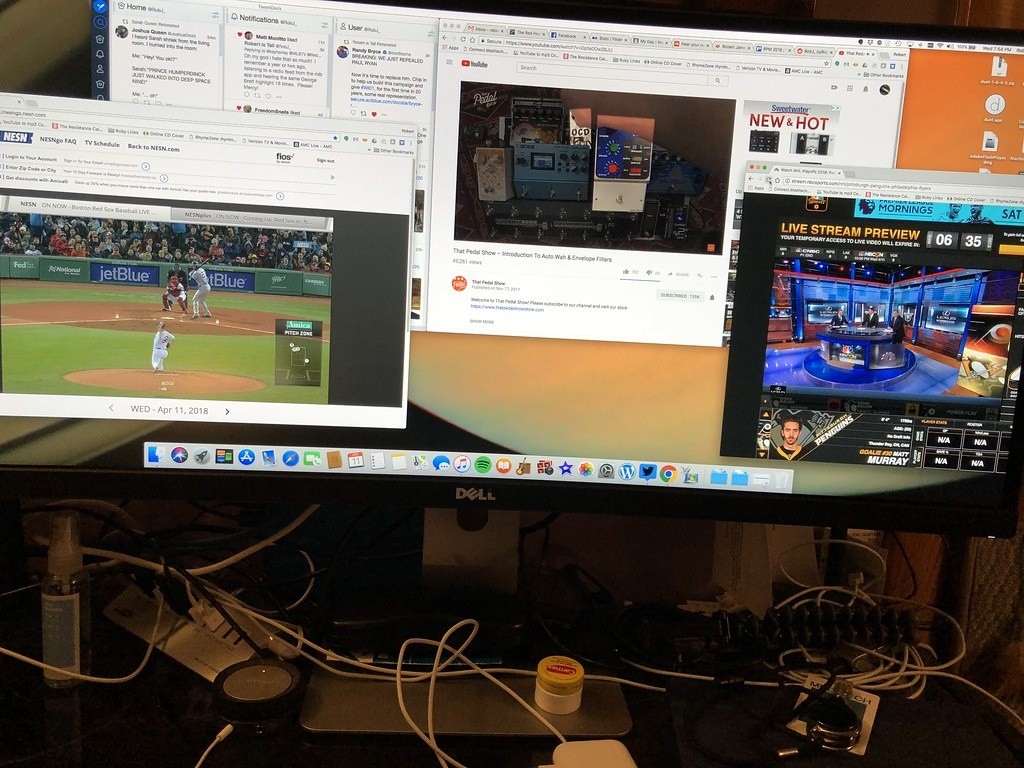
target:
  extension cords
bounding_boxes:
[101,567,290,686]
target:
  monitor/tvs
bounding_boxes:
[0,0,1024,738]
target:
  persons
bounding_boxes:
[0,211,333,273]
[769,416,803,460]
[152,322,175,374]
[161,264,189,313]
[936,203,995,224]
[889,310,906,344]
[860,308,878,330]
[188,262,212,320]
[830,309,848,326]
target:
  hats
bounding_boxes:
[19,227,26,232]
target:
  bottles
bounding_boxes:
[41,509,91,686]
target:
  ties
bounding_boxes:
[840,316,841,321]
[893,318,896,327]
[870,316,872,322]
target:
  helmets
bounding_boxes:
[170,276,179,285]
[172,264,182,274]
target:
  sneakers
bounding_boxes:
[162,308,168,311]
[185,309,188,314]
[203,313,212,317]
[190,313,200,320]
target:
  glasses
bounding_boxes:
[134,240,138,242]
[61,235,66,236]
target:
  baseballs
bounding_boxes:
[162,387,167,392]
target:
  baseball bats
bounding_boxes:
[190,257,209,274]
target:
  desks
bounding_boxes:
[815,326,896,370]
[0,623,1023,768]
